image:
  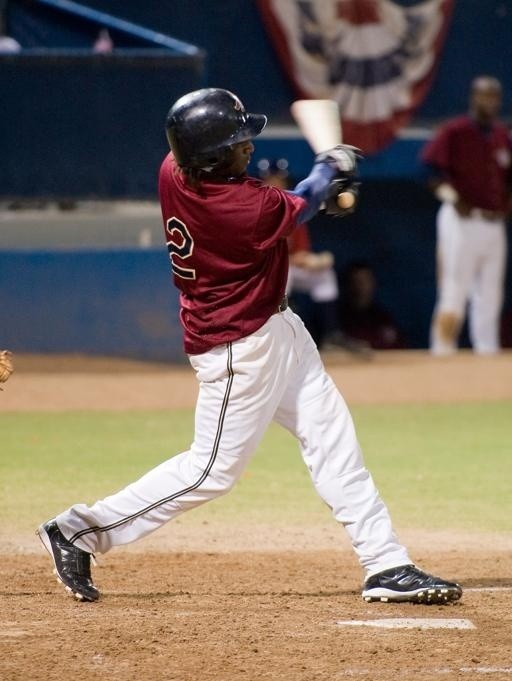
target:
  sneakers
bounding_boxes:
[361,566,464,602]
[35,516,102,601]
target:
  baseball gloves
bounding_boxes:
[0,350,15,383]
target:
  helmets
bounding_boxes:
[164,88,268,161]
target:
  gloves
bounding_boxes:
[318,139,369,216]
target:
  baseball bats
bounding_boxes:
[290,99,355,210]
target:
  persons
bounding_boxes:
[418,76,510,353]
[37,86,462,605]
[253,153,337,352]
